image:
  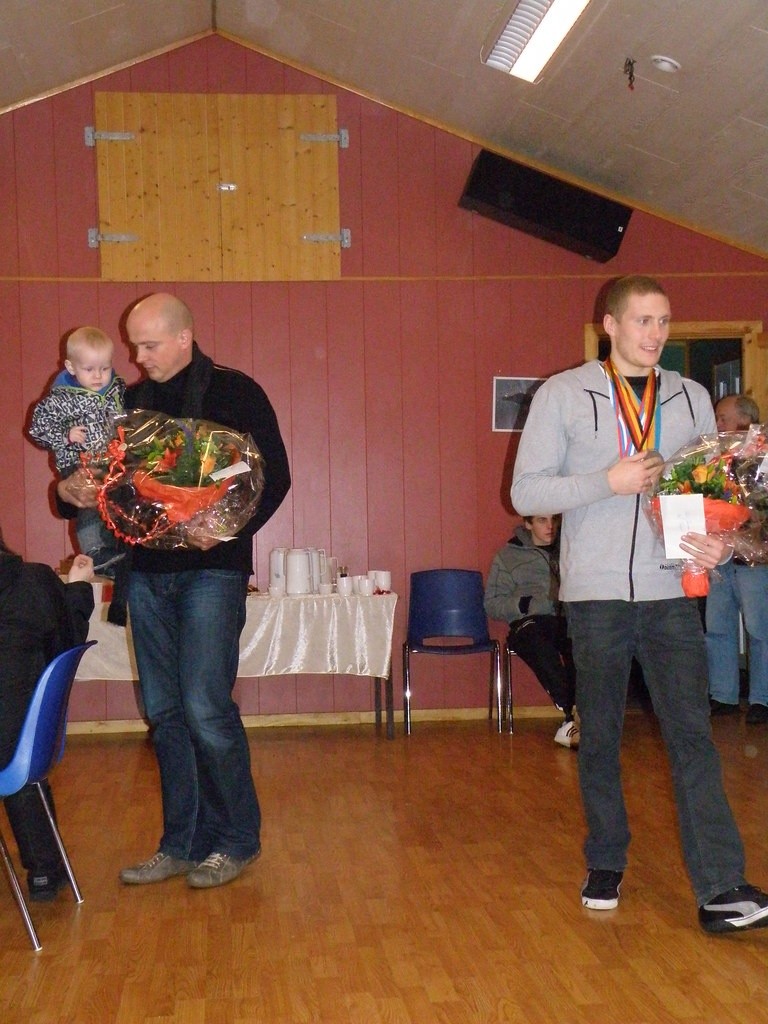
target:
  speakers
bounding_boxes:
[458,150,634,264]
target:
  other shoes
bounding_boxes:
[709,699,739,714]
[28,860,67,900]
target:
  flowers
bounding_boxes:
[641,424,768,598]
[76,408,264,548]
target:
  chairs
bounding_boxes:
[0,640,99,952]
[403,568,502,736]
[503,644,520,735]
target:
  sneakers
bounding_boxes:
[697,885,768,934]
[186,848,262,888]
[118,850,200,884]
[554,712,580,749]
[581,867,624,910]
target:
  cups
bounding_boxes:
[368,571,379,585]
[318,583,333,595]
[337,577,353,595]
[353,575,368,595]
[268,587,283,596]
[376,572,391,591]
[358,579,374,596]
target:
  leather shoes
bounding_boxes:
[747,703,768,723]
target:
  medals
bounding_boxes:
[644,450,665,475]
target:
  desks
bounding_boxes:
[75,591,398,740]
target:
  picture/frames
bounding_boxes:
[492,376,547,432]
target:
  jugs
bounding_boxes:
[270,548,287,593]
[286,549,312,594]
[308,547,326,592]
[320,554,339,586]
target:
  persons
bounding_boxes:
[60,293,291,893]
[29,327,127,568]
[0,529,94,903]
[509,275,768,933]
[484,394,768,749]
